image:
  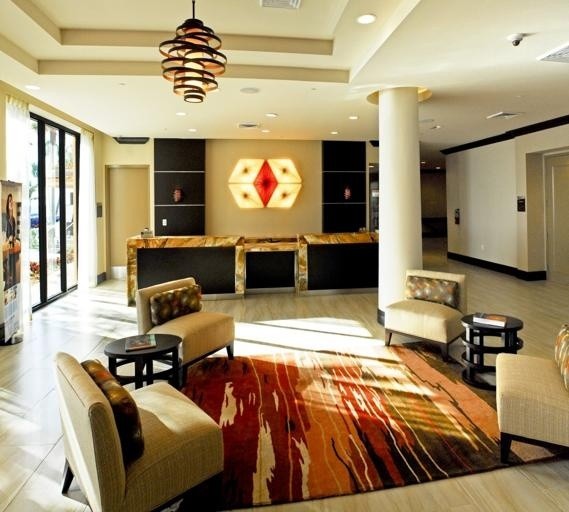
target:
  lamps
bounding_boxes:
[171,184,184,201]
[159,2,231,106]
[342,185,350,198]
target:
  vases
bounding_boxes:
[30,273,39,285]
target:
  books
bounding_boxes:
[125,334,157,352]
[472,312,507,327]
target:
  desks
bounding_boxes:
[106,333,184,391]
[458,311,525,392]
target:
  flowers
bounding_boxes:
[30,262,40,273]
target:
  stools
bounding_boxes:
[494,350,569,466]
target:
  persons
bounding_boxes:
[5,193,15,285]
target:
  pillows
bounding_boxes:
[404,275,460,310]
[78,357,146,470]
[146,284,205,326]
[551,324,569,393]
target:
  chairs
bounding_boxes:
[133,276,236,385]
[53,349,227,512]
[381,267,470,364]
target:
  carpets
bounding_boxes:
[169,340,569,511]
